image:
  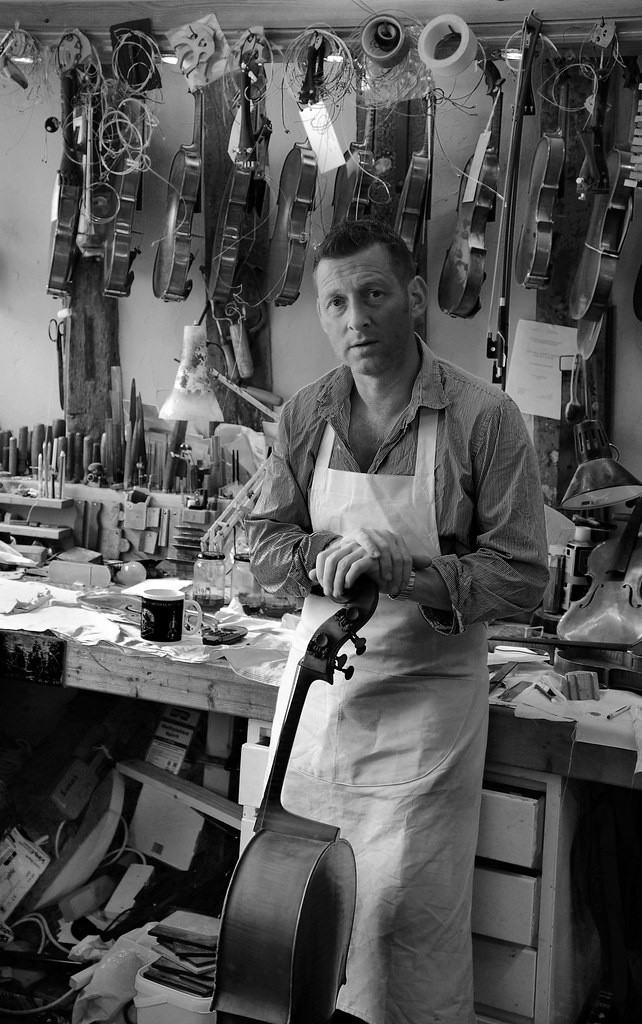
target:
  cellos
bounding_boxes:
[210,567,382,1024]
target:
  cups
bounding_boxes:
[142,589,202,646]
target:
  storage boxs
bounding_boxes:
[132,963,217,1024]
[14,695,146,820]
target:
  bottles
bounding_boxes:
[194,551,225,611]
[262,588,295,618]
[561,527,595,613]
[231,553,262,615]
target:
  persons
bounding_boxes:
[246,220,550,1024]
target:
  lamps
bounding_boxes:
[158,303,224,422]
[560,354,642,510]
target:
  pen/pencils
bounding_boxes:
[607,703,632,720]
[534,683,558,704]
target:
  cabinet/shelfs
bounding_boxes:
[238,719,572,1024]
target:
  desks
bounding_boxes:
[0,576,642,788]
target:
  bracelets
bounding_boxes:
[387,567,416,601]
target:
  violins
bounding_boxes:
[153,34,325,306]
[514,51,571,291]
[436,46,503,318]
[567,53,642,320]
[330,69,380,234]
[396,77,433,252]
[555,498,642,647]
[45,32,148,300]
